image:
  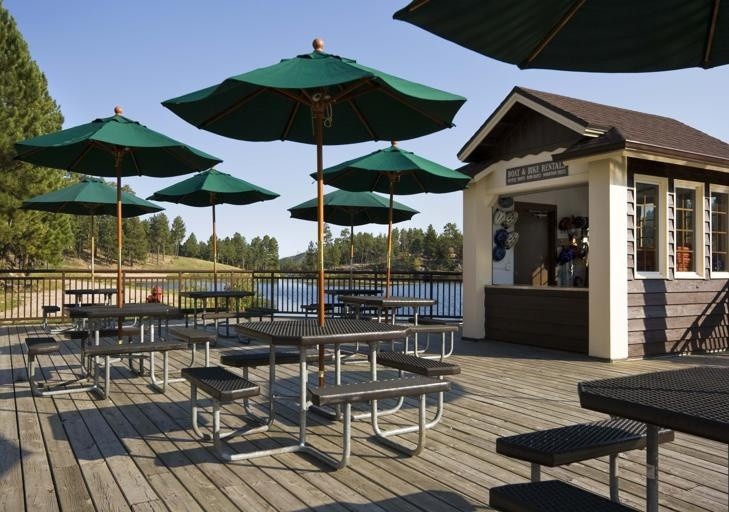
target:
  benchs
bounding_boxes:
[495,418,674,504]
[489,480,645,512]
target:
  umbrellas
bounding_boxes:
[7,105,229,345]
[18,178,167,305]
[144,165,279,310]
[308,139,471,322]
[158,46,475,388]
[286,189,419,292]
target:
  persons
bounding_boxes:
[392,1,729,72]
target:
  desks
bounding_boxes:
[577,366,729,512]
[337,296,435,358]
[66,289,118,332]
[180,291,256,338]
[324,290,384,319]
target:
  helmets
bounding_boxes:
[493,197,520,260]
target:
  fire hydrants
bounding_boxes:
[143,284,162,302]
